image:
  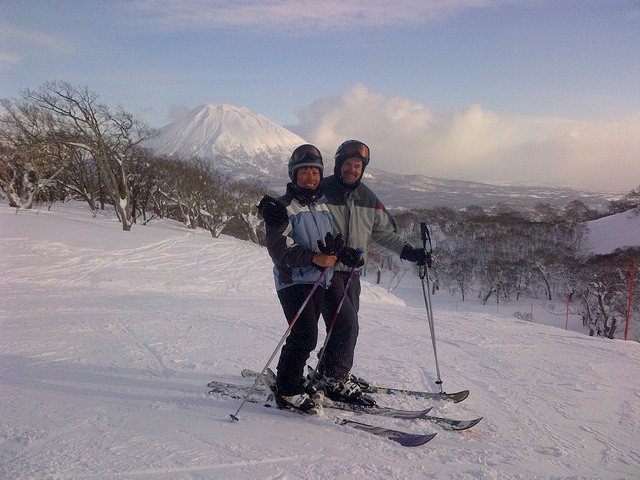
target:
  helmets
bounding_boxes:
[335,140,370,174]
[288,145,324,182]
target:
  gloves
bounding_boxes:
[313,232,344,272]
[258,195,289,228]
[339,246,364,268]
[400,243,432,268]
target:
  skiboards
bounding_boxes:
[207,381,437,447]
[241,368,484,431]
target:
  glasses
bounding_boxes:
[336,143,369,163]
[288,145,323,167]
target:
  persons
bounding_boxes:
[254,139,433,399]
[264,143,378,412]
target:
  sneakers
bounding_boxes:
[275,383,310,407]
[344,371,369,391]
[336,370,362,397]
[323,371,357,403]
